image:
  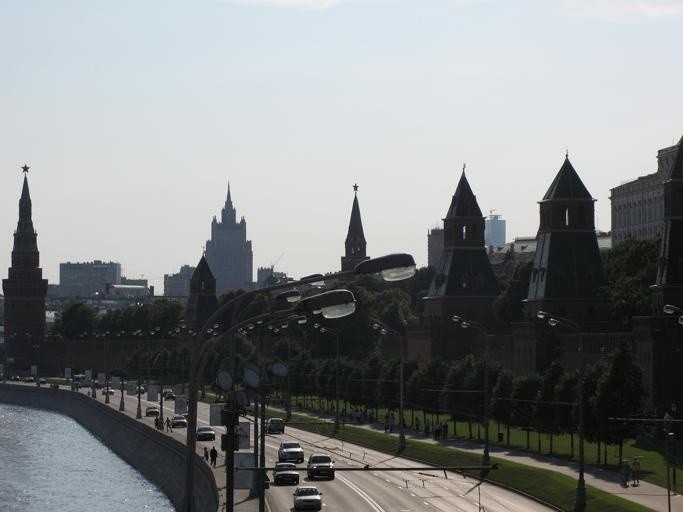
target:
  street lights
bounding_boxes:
[668,429,676,491]
[661,301,683,324]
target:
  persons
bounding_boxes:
[160,420,165,432]
[164,417,172,433]
[209,447,217,467]
[349,407,447,441]
[153,416,158,429]
[631,458,640,485]
[203,446,208,461]
[623,461,630,485]
